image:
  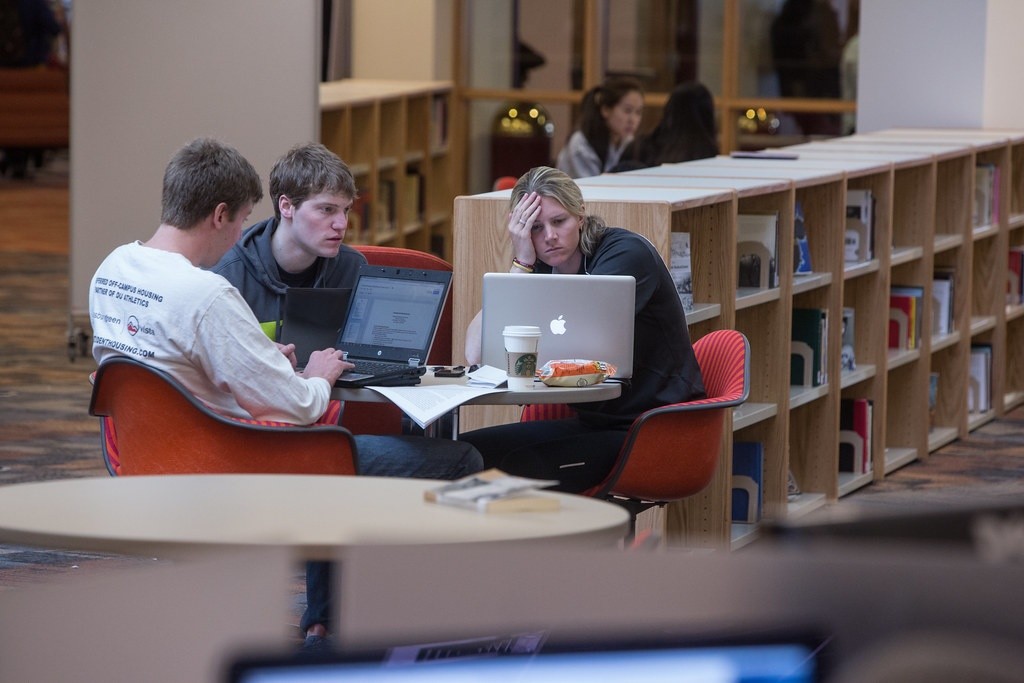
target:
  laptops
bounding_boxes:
[295,263,451,386]
[479,272,635,379]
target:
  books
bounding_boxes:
[668,155,1024,526]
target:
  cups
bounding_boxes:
[502,326,542,391]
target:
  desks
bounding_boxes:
[1,476,632,650]
[289,364,622,436]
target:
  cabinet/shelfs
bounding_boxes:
[449,130,1024,548]
[313,78,461,263]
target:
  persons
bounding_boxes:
[0,0,68,182]
[465,166,706,494]
[769,0,845,140]
[609,82,715,172]
[206,142,376,648]
[89,136,485,481]
[555,76,643,179]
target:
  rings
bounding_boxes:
[519,220,526,225]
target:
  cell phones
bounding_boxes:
[434,369,465,377]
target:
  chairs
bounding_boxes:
[519,330,754,551]
[349,243,451,368]
[89,356,360,476]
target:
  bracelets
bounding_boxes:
[512,258,535,273]
